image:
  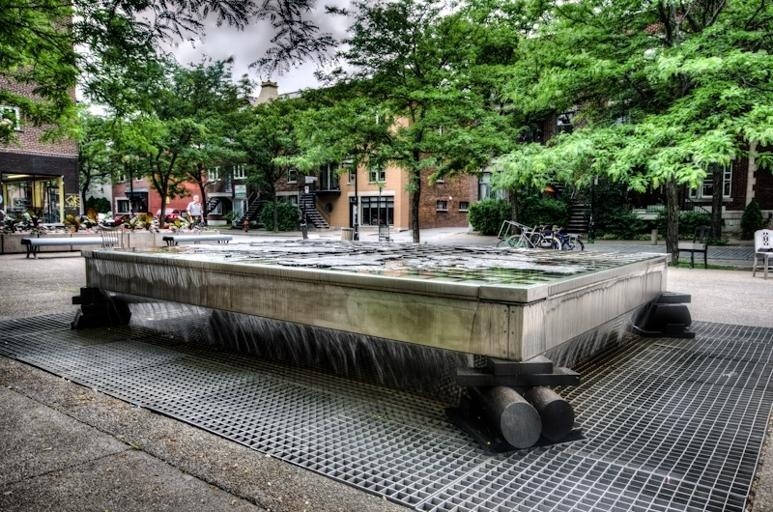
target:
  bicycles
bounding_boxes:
[496,219,584,252]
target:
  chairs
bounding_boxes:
[677,225,711,268]
[753,229,773,279]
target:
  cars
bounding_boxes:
[156,208,189,222]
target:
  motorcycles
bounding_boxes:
[0,207,208,233]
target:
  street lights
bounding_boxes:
[347,148,361,241]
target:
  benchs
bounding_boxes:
[21,235,118,259]
[163,234,232,246]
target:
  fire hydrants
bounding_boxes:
[242,219,251,232]
